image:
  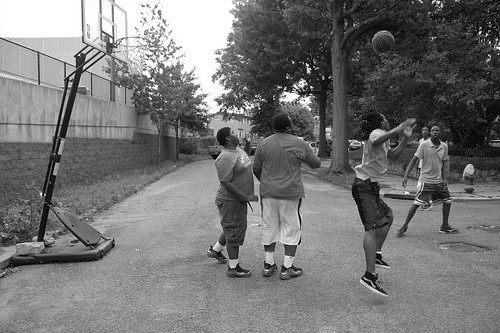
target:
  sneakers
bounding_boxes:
[207,245,227,263]
[439,225,460,234]
[262,261,278,277]
[227,262,251,278]
[395,225,408,236]
[374,253,391,269]
[360,271,388,297]
[280,264,303,279]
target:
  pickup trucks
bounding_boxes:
[208,142,244,159]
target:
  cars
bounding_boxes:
[306,141,318,155]
[348,139,362,151]
[483,134,500,148]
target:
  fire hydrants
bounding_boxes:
[464,172,477,185]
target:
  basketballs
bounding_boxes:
[372,30,394,53]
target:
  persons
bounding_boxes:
[395,123,459,236]
[350,108,418,297]
[206,125,259,277]
[416,126,435,212]
[253,112,321,280]
